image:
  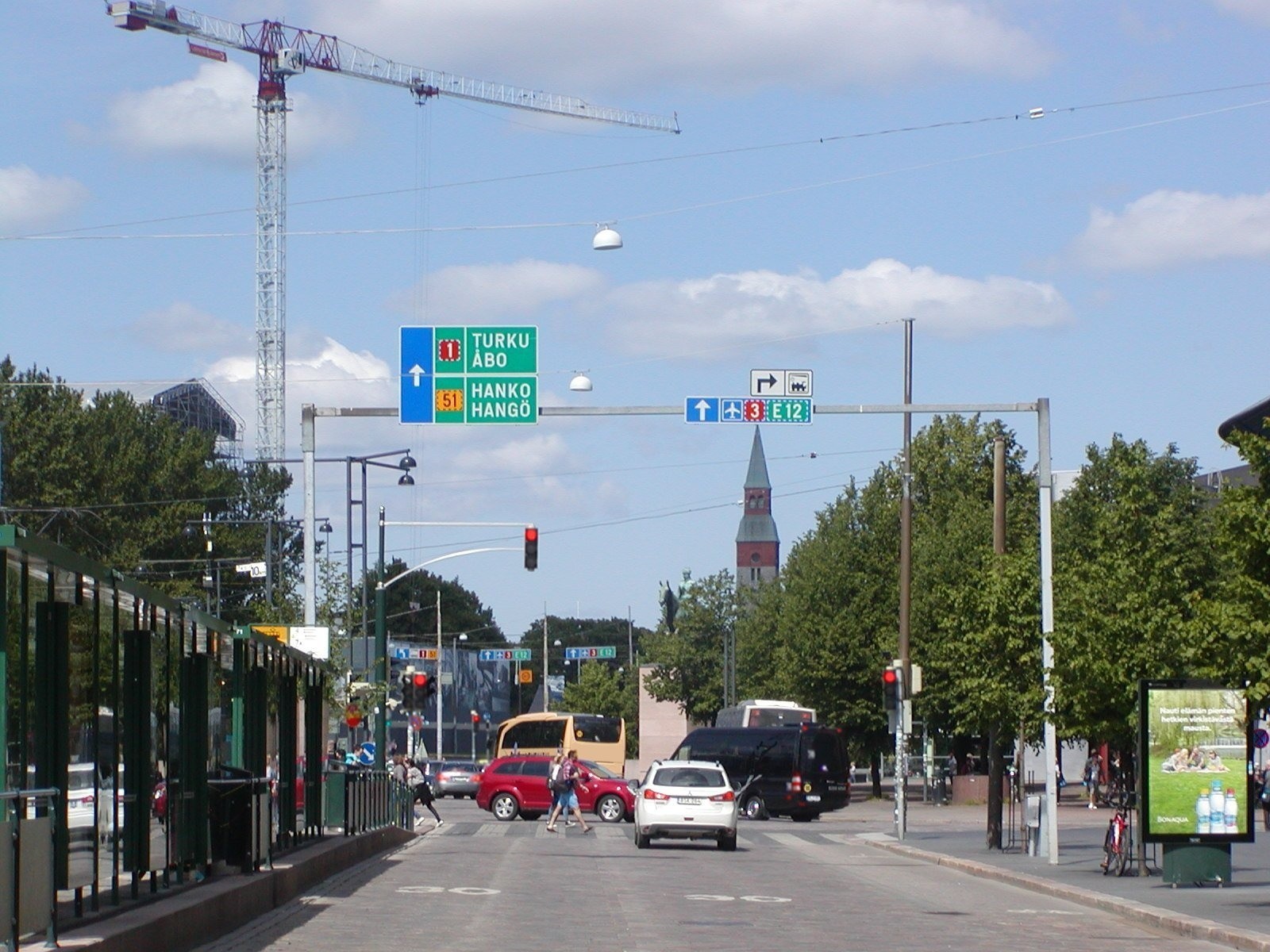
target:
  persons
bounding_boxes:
[1083,748,1122,809]
[1162,747,1230,771]
[545,750,594,833]
[947,751,974,785]
[1258,760,1270,830]
[849,762,856,784]
[1055,757,1062,805]
[330,744,444,827]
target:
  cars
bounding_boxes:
[155,780,169,826]
[274,752,306,816]
[417,760,442,793]
[436,760,483,800]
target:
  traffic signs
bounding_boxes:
[400,325,538,424]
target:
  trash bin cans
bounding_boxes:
[326,770,345,828]
[207,778,272,876]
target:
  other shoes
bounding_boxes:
[1088,803,1094,810]
[546,822,557,828]
[547,828,557,833]
[565,821,575,828]
[415,816,425,827]
[436,820,444,828]
[1095,805,1098,810]
[584,827,594,833]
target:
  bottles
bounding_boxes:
[1195,779,1237,834]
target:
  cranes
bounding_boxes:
[106,0,681,522]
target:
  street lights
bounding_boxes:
[347,447,418,751]
[266,516,332,610]
[182,520,213,616]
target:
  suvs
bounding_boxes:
[25,761,126,845]
[627,758,741,851]
[476,754,635,823]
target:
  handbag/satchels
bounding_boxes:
[417,782,436,805]
[547,776,556,789]
[1059,780,1067,787]
[1261,792,1270,803]
[1084,769,1092,783]
[554,767,575,794]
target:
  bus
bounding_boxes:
[496,711,626,779]
[715,699,816,729]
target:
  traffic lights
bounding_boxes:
[881,668,898,710]
[402,674,412,710]
[524,527,538,571]
[413,672,428,710]
[472,716,479,734]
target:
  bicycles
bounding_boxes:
[1100,802,1130,877]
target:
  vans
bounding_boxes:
[645,726,851,822]
